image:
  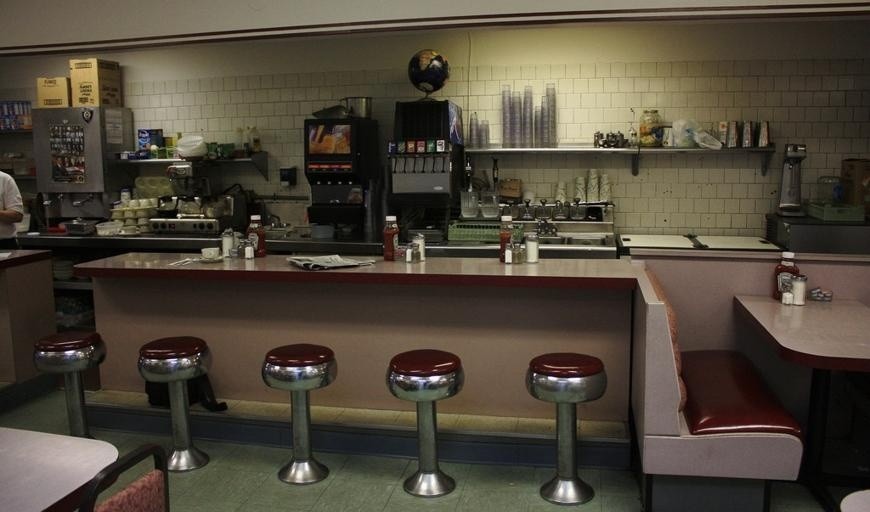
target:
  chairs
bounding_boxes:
[78,443,170,512]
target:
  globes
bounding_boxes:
[407,48,454,100]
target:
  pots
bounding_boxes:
[340,97,372,118]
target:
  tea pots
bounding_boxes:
[157,194,176,212]
[203,198,230,218]
[174,194,202,216]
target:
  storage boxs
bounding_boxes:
[841,158,870,205]
[36,78,71,108]
[70,58,123,108]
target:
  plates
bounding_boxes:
[200,256,222,262]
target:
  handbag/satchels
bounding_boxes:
[145,373,207,407]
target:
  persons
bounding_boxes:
[0,171,24,249]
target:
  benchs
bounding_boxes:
[632,260,805,512]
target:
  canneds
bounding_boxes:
[640,109,662,147]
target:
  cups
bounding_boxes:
[467,111,490,150]
[458,189,480,219]
[479,189,501,219]
[554,166,613,204]
[202,248,220,259]
[501,82,559,148]
[111,198,157,234]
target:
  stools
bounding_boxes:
[262,342,337,484]
[137,337,209,471]
[524,352,608,506]
[33,334,107,439]
[385,349,464,498]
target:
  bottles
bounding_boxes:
[222,215,265,259]
[638,108,664,147]
[0,101,33,129]
[499,216,540,263]
[593,131,624,148]
[789,274,808,306]
[773,252,800,300]
[727,120,770,148]
[234,126,261,152]
[383,215,426,264]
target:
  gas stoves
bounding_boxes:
[149,215,220,234]
